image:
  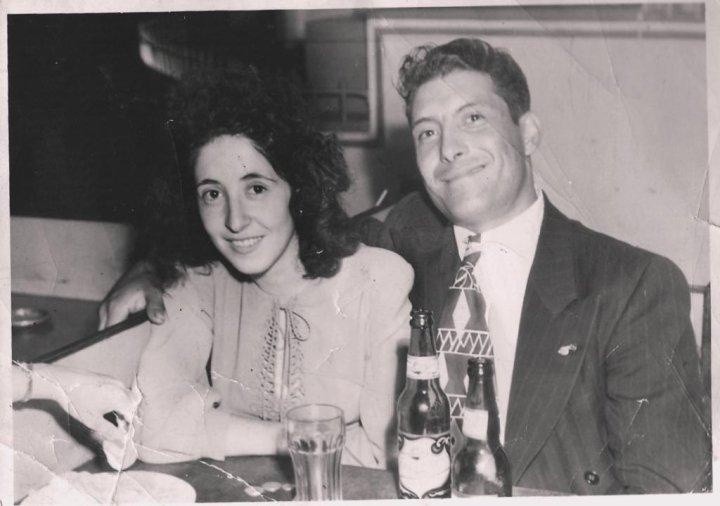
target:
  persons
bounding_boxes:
[10,362,134,441]
[131,106,414,464]
[97,37,712,498]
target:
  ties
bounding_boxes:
[453,237,485,352]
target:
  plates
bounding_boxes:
[19,470,197,505]
[11,304,50,330]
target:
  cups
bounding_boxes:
[284,399,347,502]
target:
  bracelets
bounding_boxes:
[12,361,33,403]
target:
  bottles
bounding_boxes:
[397,309,453,499]
[454,358,512,496]
